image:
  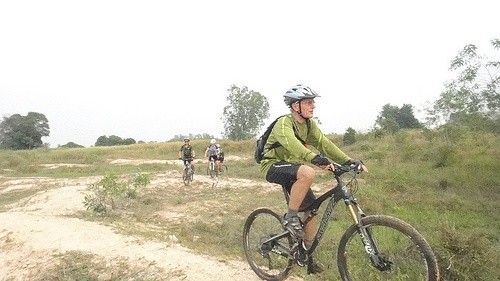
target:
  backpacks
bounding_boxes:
[255,115,311,164]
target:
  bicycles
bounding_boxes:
[207,155,228,178]
[242,160,439,281]
[181,156,194,186]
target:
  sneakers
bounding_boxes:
[296,251,325,273]
[281,213,304,239]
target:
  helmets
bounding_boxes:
[215,144,220,148]
[282,85,318,107]
[211,139,216,144]
[184,138,191,141]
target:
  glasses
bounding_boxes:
[294,98,315,107]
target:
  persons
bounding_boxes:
[178,138,195,169]
[260,84,369,275]
[204,139,224,177]
